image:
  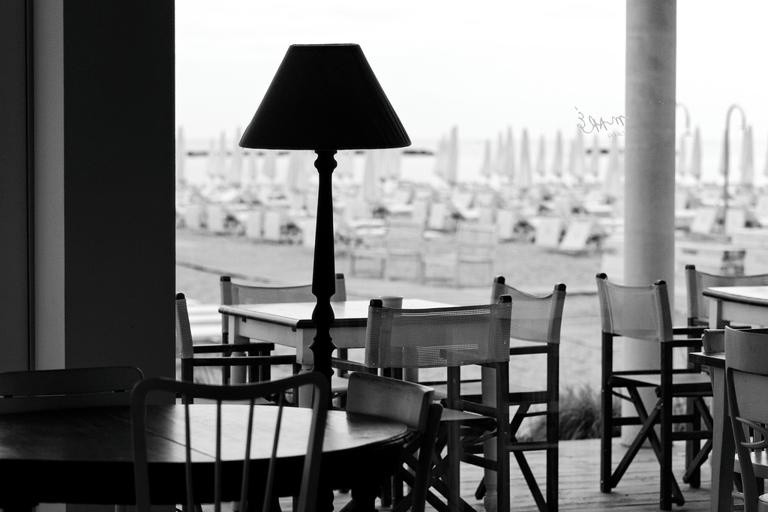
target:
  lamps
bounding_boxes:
[238,44,412,412]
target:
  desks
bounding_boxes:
[700,285,767,334]
[687,350,767,512]
[2,399,415,512]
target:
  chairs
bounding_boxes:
[4,364,145,411]
[221,270,346,384]
[718,322,767,512]
[175,292,300,404]
[459,275,568,511]
[128,365,331,512]
[684,266,767,490]
[591,270,718,510]
[368,294,512,512]
[348,373,434,512]
